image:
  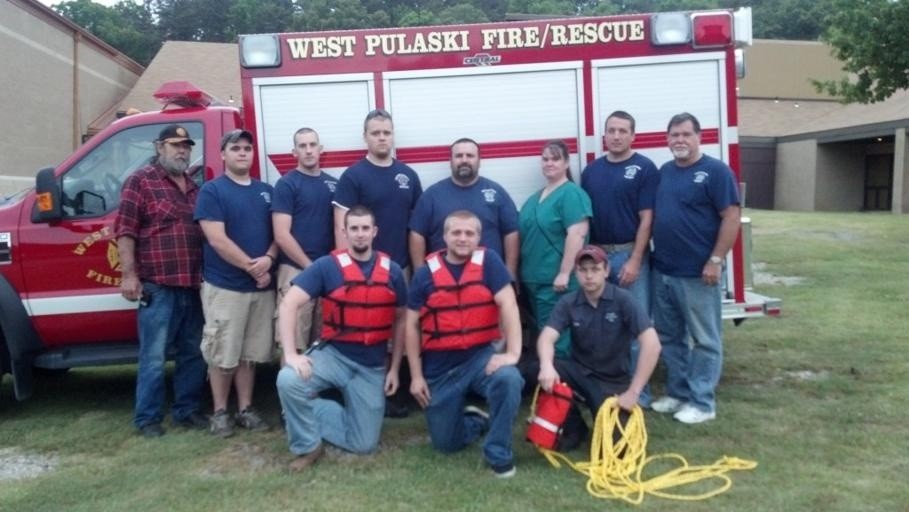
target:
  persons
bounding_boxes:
[332,109,423,418]
[648,113,740,423]
[192,129,279,438]
[404,208,524,480]
[113,126,207,439]
[270,127,337,370]
[518,138,593,358]
[580,111,660,413]
[536,244,664,465]
[410,138,523,295]
[274,206,408,473]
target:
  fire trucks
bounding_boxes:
[0,5,784,404]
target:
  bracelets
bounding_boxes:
[260,251,277,266]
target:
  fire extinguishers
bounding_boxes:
[526,367,586,450]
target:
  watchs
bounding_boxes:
[706,254,727,269]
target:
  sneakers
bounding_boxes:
[649,396,683,414]
[463,406,490,421]
[182,408,272,438]
[674,405,715,424]
[490,463,516,479]
[141,423,164,438]
[289,443,324,473]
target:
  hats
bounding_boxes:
[221,129,252,150]
[574,245,609,265]
[159,125,195,145]
